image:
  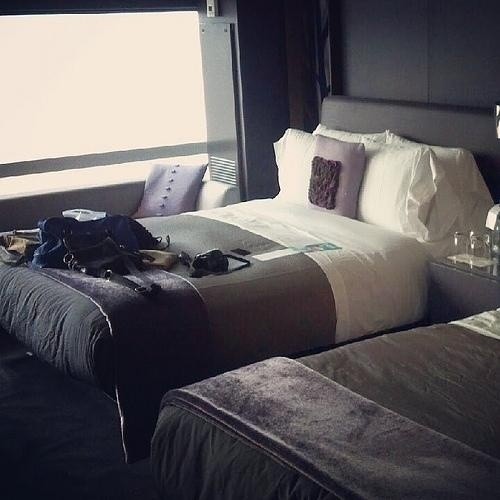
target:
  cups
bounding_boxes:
[452,229,492,267]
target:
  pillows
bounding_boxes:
[270,124,488,242]
[129,158,210,219]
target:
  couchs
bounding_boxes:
[2,177,240,232]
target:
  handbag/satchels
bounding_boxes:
[190,247,251,278]
[63,231,143,277]
[32,212,159,269]
[6,230,40,261]
[133,249,180,272]
